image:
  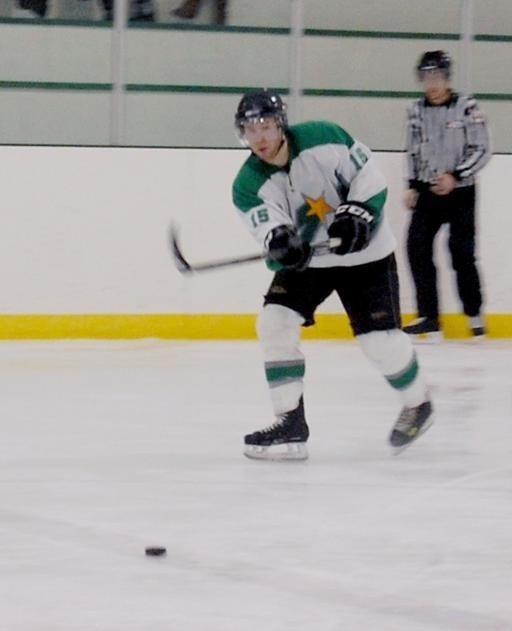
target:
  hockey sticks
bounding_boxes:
[169,223,333,274]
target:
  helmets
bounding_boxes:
[234,91,288,131]
[418,51,450,74]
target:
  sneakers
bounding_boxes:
[470,314,484,336]
[402,319,438,334]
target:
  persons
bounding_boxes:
[399,47,497,337]
[229,89,435,449]
[168,0,229,25]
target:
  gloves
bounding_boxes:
[264,224,313,274]
[325,203,375,256]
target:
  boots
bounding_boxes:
[389,402,432,446]
[243,394,311,447]
